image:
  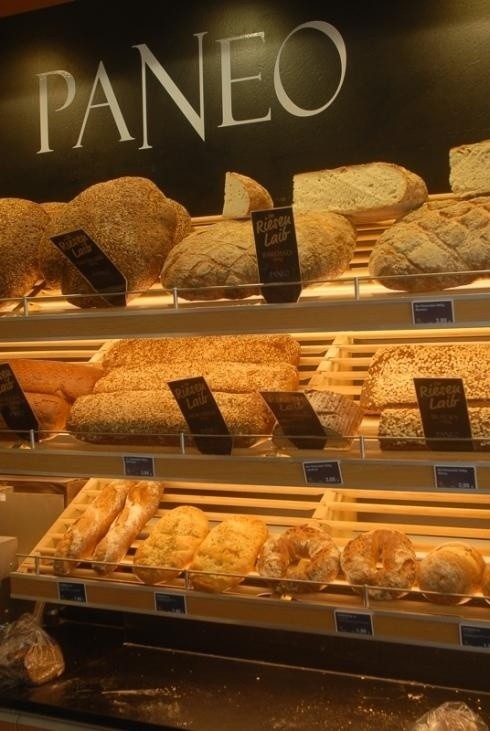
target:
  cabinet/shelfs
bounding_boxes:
[0,187,490,729]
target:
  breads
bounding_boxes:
[100,335,300,365]
[294,161,425,224]
[158,211,352,299]
[0,357,101,396]
[272,387,362,448]
[446,140,490,196]
[54,478,135,568]
[93,365,300,393]
[134,506,210,584]
[253,523,340,596]
[63,392,272,442]
[416,541,484,604]
[7,643,63,687]
[188,517,268,591]
[361,345,490,412]
[341,528,417,600]
[40,176,191,309]
[377,406,490,451]
[368,201,489,289]
[91,480,164,575]
[223,170,273,218]
[0,198,75,311]
[0,393,77,430]
